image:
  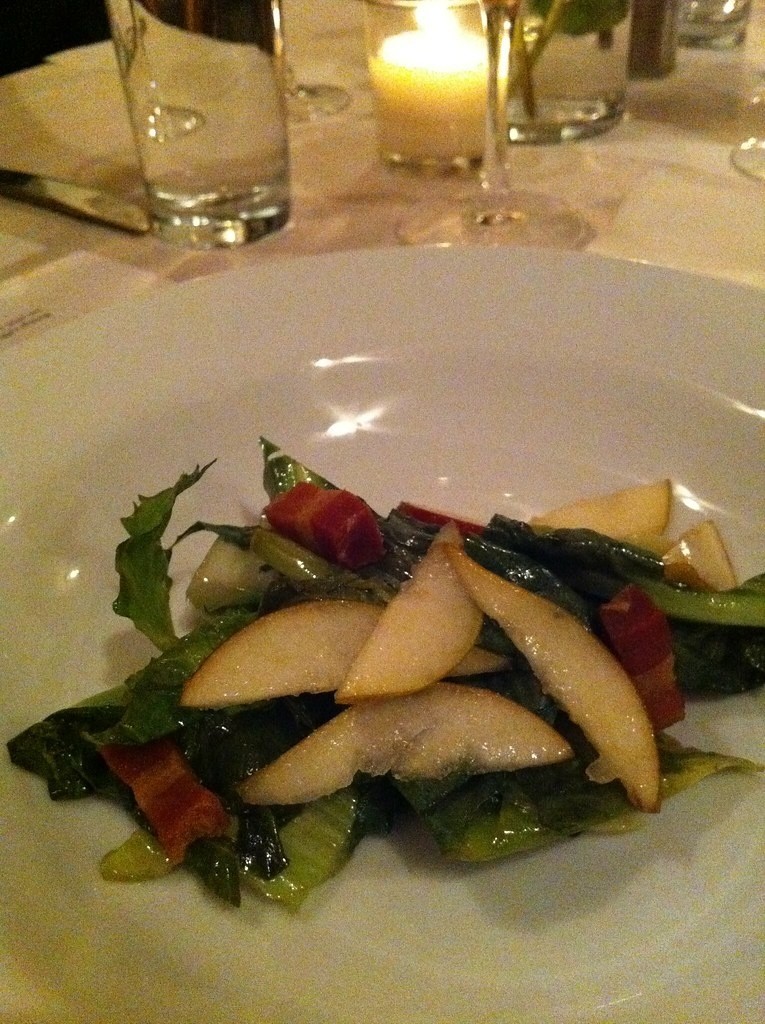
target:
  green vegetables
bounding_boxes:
[5,435,765,908]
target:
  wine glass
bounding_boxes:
[272,2,350,127]
[392,0,589,252]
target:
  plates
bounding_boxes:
[0,245,765,1023]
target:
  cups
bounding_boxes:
[360,0,509,179]
[671,0,757,50]
[498,0,638,143]
[99,0,295,246]
[731,1,765,183]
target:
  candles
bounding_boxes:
[371,18,490,165]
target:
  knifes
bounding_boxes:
[0,169,155,231]
